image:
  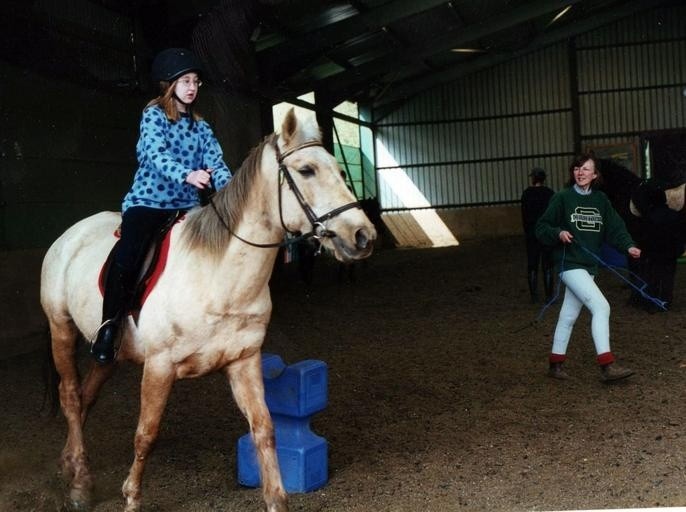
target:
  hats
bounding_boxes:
[527,167,546,178]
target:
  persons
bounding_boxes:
[91,46,235,366]
[519,166,559,307]
[531,151,644,387]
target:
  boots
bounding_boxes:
[544,271,556,305]
[527,271,540,304]
[92,270,136,365]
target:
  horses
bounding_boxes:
[39,105,378,512]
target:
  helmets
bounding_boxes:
[149,48,202,82]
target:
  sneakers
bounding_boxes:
[545,360,569,380]
[595,363,638,382]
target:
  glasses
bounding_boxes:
[177,78,204,89]
